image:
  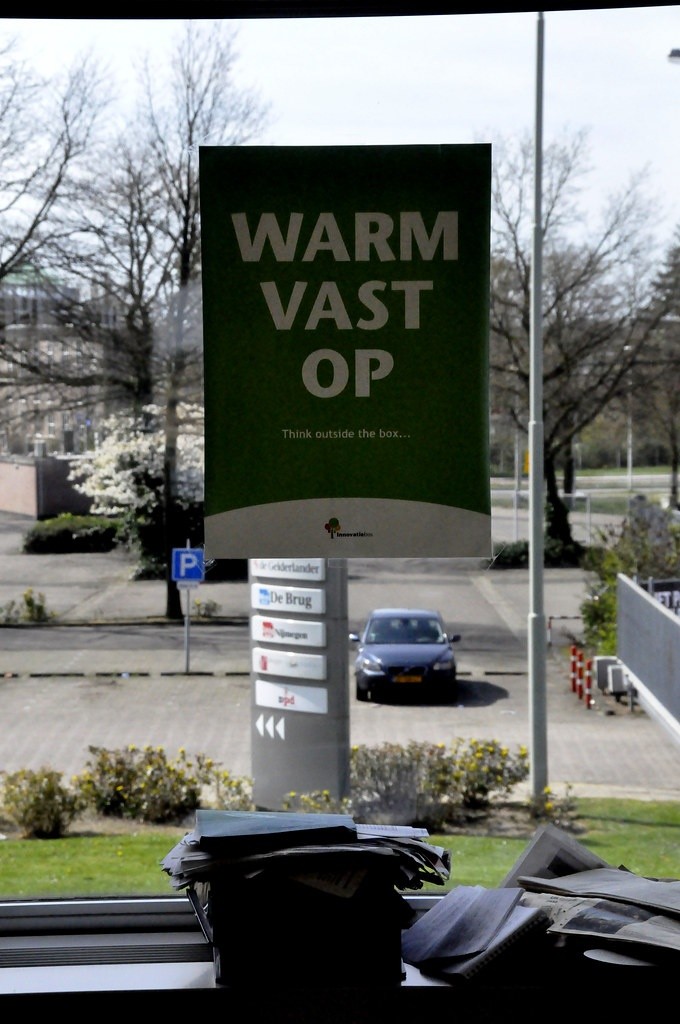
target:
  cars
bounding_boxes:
[349,609,461,700]
